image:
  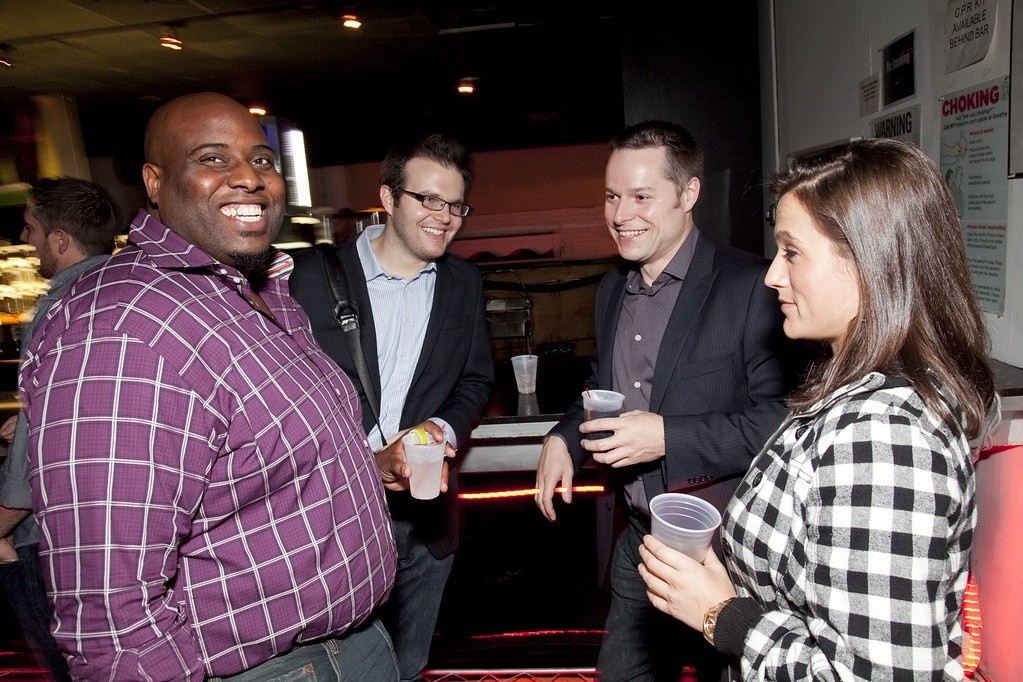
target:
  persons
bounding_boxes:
[534,120,797,682]
[638,136,996,682]
[0,174,115,682]
[19,89,455,682]
[289,130,494,682]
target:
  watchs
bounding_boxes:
[703,597,736,646]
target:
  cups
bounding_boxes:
[401,431,447,500]
[581,390,626,440]
[650,493,722,565]
[510,354,539,394]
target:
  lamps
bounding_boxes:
[340,15,362,29]
[456,77,479,93]
[159,23,189,50]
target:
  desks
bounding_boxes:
[460,411,629,630]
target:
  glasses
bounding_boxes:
[397,188,470,217]
